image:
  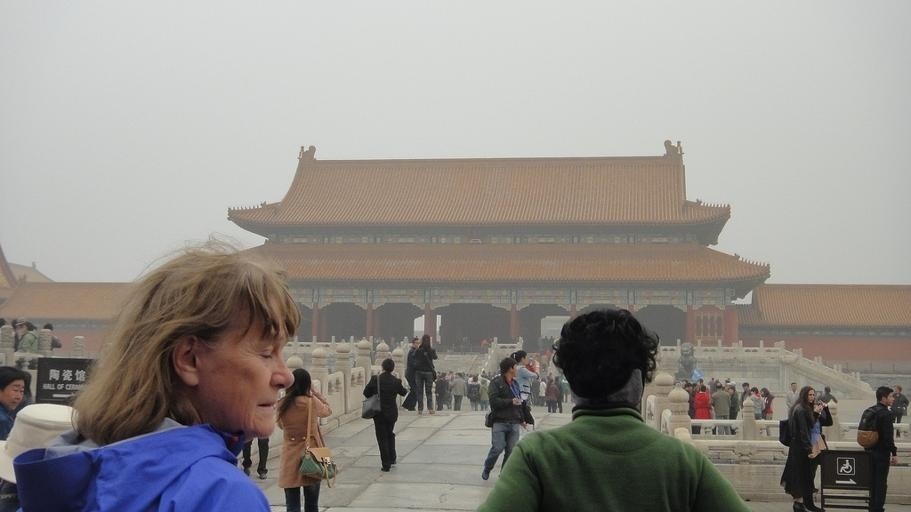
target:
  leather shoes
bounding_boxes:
[793,501,812,511]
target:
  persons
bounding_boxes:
[859,386,897,512]
[0,317,61,370]
[820,385,838,404]
[364,359,407,472]
[0,366,24,442]
[277,364,331,512]
[436,336,569,485]
[403,334,438,414]
[782,386,825,512]
[240,436,270,479]
[892,386,909,437]
[1,253,330,512]
[681,377,773,436]
[477,312,771,510]
[786,383,800,417]
[812,398,833,492]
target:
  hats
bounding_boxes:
[1,404,79,484]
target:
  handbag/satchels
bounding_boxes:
[778,420,791,447]
[432,370,437,381]
[485,412,495,428]
[299,447,339,488]
[361,393,382,419]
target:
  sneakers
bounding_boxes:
[482,468,489,480]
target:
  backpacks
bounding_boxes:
[856,406,889,449]
